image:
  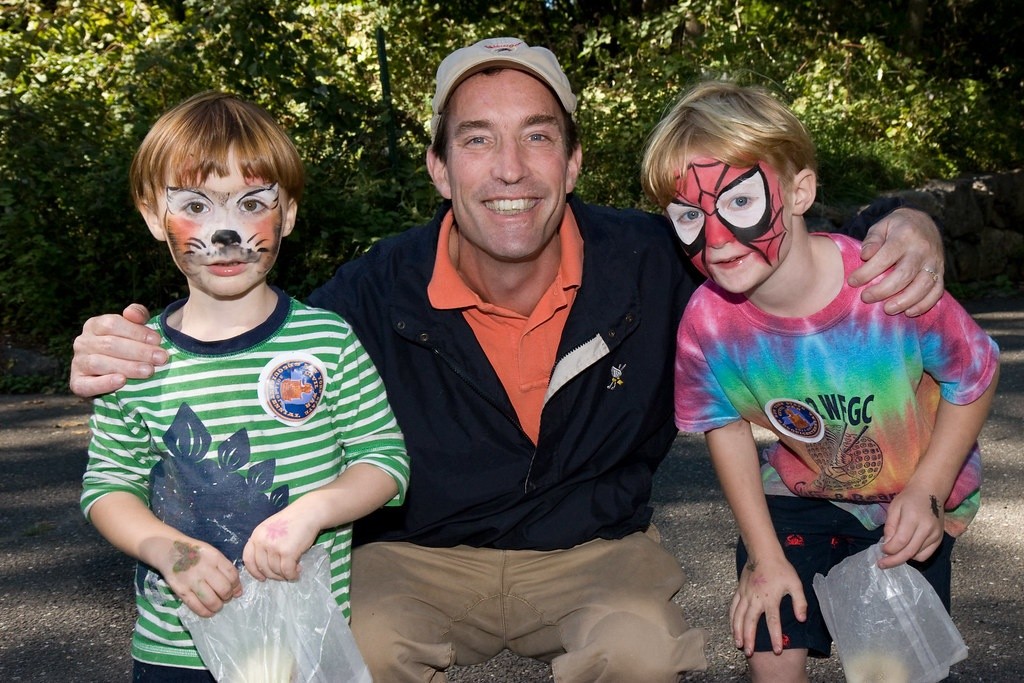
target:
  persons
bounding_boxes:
[80,89,410,683]
[639,82,1001,683]
[69,37,950,683]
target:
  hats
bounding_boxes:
[430,37,577,145]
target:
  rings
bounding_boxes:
[923,268,939,284]
